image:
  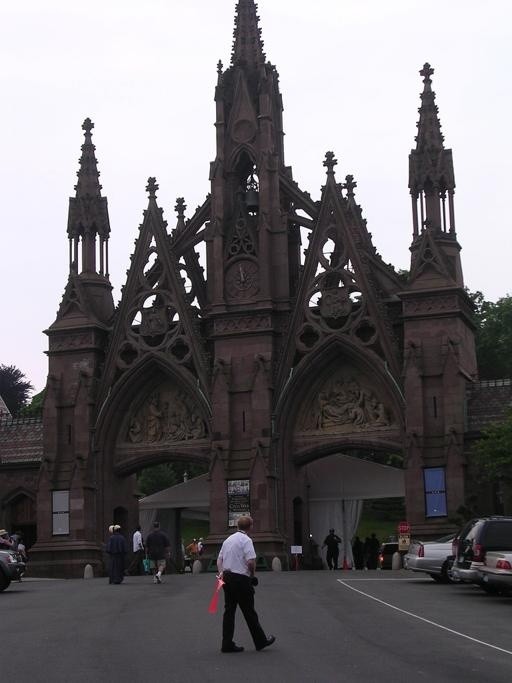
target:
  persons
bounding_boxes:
[124,526,146,576]
[322,528,343,569]
[146,520,171,583]
[9,529,23,546]
[351,532,380,569]
[126,387,207,441]
[107,524,129,584]
[19,539,29,576]
[180,537,206,573]
[0,529,14,550]
[316,374,388,429]
[216,514,275,652]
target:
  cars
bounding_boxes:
[379,543,399,570]
[0,550,27,592]
[403,516,512,594]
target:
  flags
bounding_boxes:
[209,577,226,615]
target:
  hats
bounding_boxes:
[0,529,8,536]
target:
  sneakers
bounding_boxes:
[153,572,165,584]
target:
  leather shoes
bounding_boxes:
[220,644,246,653]
[256,633,276,652]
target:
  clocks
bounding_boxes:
[223,255,261,300]
